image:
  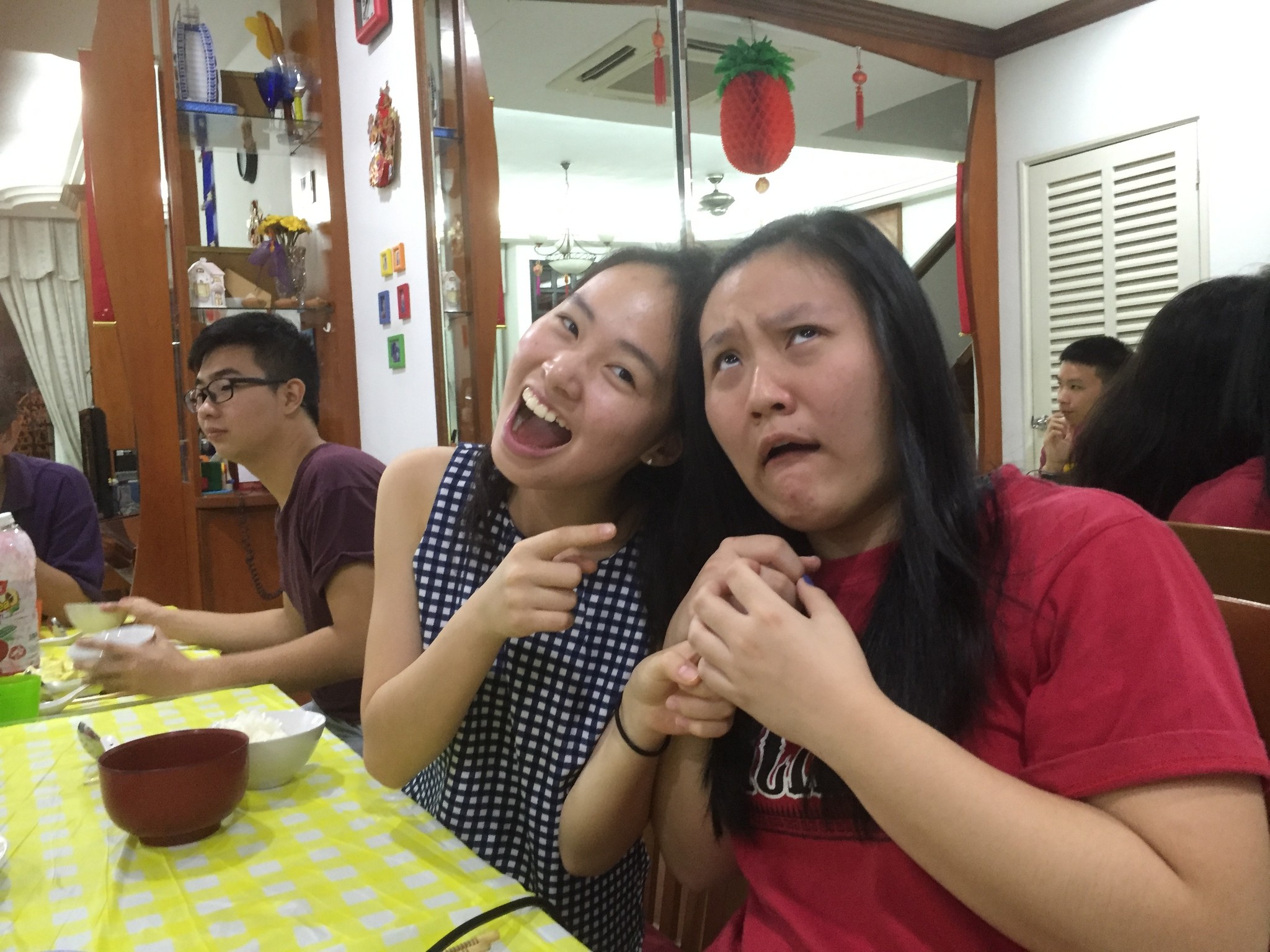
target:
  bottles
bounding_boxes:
[0,511,41,677]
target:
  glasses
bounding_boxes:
[184,377,305,413]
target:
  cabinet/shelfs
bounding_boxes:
[177,108,330,314]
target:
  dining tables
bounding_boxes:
[0,602,594,951]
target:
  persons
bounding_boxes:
[72,309,386,756]
[650,204,1270,952]
[0,373,103,630]
[360,244,718,952]
[1038,265,1270,534]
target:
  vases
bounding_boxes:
[271,248,309,312]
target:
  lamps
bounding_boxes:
[696,173,736,216]
[531,161,614,275]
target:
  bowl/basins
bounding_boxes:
[97,727,250,848]
[65,624,156,669]
[211,709,326,791]
[63,601,128,635]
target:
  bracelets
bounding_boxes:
[615,695,671,755]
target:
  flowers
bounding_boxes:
[253,211,312,266]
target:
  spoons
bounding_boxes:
[38,683,91,717]
[38,630,83,646]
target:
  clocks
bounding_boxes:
[353,0,390,45]
